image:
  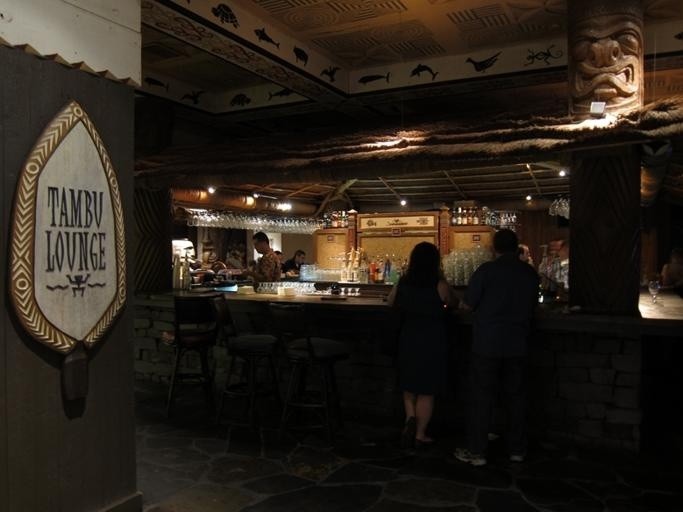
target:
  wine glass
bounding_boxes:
[441,248,491,281]
[548,197,569,220]
[647,280,661,307]
[185,210,320,235]
[255,282,316,294]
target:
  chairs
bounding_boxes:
[165,292,238,417]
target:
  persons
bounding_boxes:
[518,244,535,266]
[194,232,306,287]
[387,242,451,443]
[661,246,683,289]
[454,229,541,466]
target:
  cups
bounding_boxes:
[193,277,199,284]
[299,264,341,281]
[284,287,294,296]
[225,271,232,281]
[278,287,284,296]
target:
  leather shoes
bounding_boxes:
[416,437,436,447]
[398,415,415,442]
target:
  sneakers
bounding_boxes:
[453,446,485,466]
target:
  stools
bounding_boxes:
[215,332,280,452]
[282,336,351,462]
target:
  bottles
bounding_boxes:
[341,254,408,283]
[322,211,348,229]
[182,256,190,288]
[538,252,568,302]
[450,206,516,230]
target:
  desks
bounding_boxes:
[295,279,469,299]
